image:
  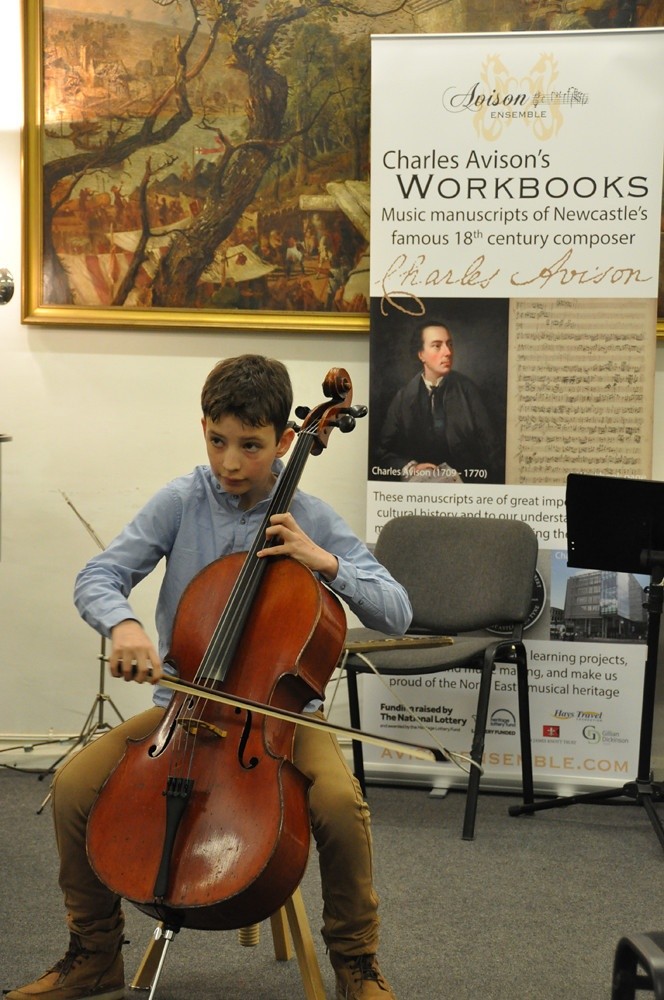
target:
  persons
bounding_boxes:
[0,352,415,1000]
[388,321,504,483]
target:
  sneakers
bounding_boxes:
[3,933,131,1000]
[329,950,396,1000]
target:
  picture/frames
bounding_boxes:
[21,0,664,341]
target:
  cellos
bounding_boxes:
[82,366,372,1000]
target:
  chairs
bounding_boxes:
[335,514,538,841]
